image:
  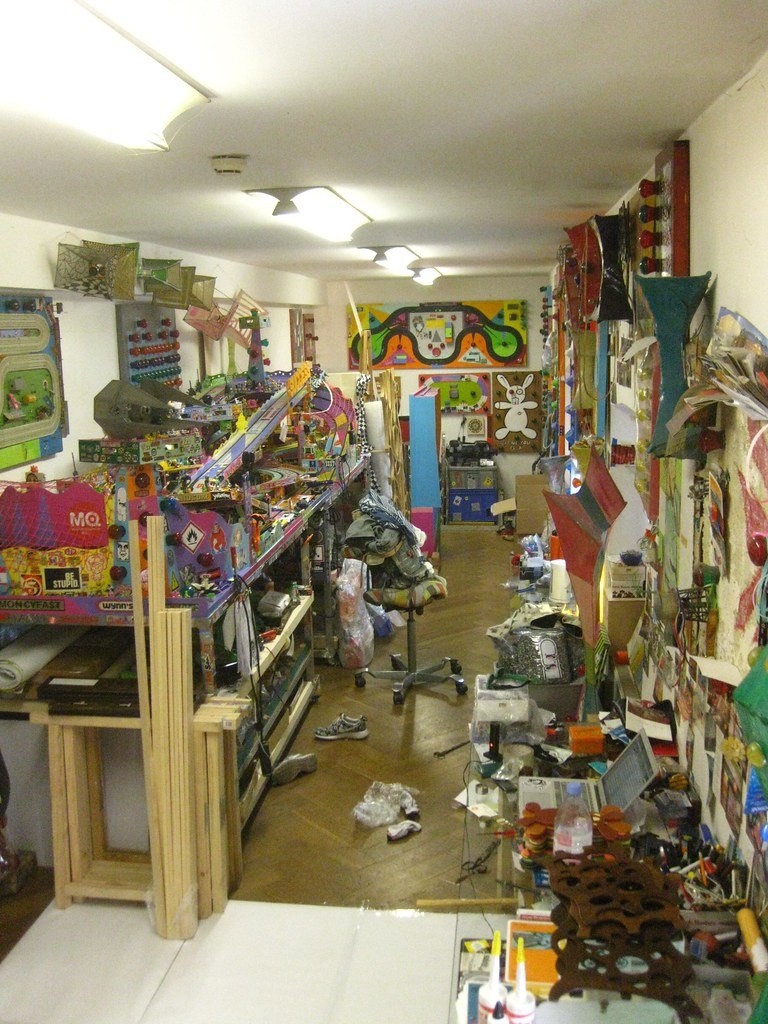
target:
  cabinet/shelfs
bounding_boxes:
[0,454,370,872]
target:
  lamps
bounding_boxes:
[548,559,572,605]
[0,0,209,152]
[358,246,442,286]
[244,185,372,243]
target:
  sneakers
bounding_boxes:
[271,752,317,786]
[314,712,368,740]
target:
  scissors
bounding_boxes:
[460,837,502,874]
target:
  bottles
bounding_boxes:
[487,1001,509,1024]
[477,930,506,1024]
[508,938,535,1024]
[554,780,594,856]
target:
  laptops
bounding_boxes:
[519,729,659,820]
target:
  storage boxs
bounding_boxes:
[510,554,545,581]
[491,475,551,535]
[258,590,290,615]
[410,507,434,558]
[602,555,646,651]
[449,466,497,489]
[79,434,201,464]
[529,680,584,721]
[448,487,499,524]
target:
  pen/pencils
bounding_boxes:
[677,853,736,897]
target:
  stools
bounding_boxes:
[355,578,469,706]
